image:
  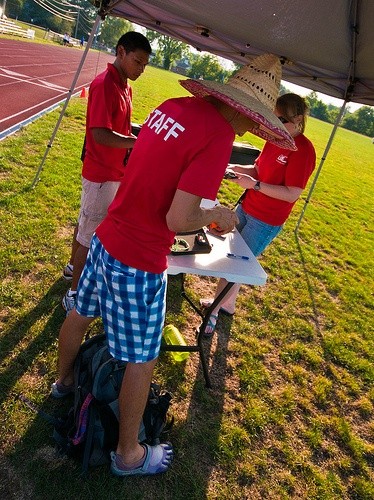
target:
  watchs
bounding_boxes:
[254,182,261,191]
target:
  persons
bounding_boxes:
[61,31,152,317]
[63,32,71,47]
[197,94,315,336]
[49,54,297,475]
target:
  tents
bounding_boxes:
[31,0,373,233]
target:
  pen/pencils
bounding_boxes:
[207,230,226,239]
[227,253,249,260]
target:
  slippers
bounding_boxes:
[53,379,76,397]
[196,313,218,336]
[110,439,173,475]
[199,298,236,316]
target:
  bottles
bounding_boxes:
[163,323,191,361]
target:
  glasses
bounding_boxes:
[278,113,303,125]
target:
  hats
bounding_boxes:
[179,53,298,152]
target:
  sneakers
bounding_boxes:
[62,289,78,316]
[64,265,76,279]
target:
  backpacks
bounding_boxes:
[55,322,170,470]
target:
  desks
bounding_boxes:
[160,197,267,390]
